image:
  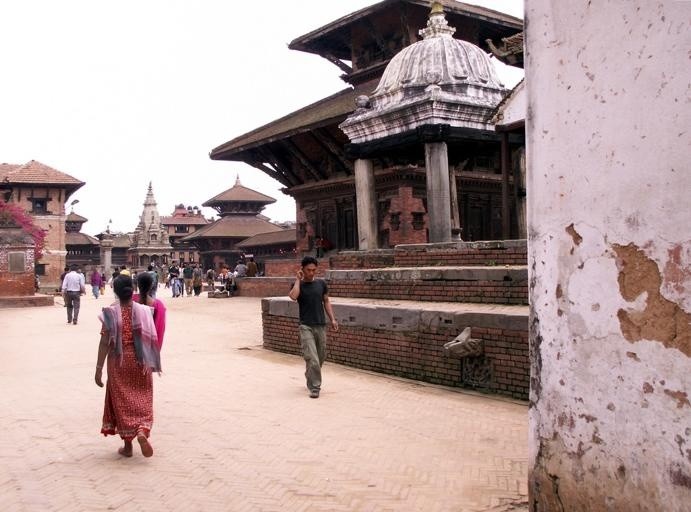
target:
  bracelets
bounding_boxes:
[95,366,102,370]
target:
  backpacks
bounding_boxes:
[208,271,213,279]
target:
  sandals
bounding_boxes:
[119,448,133,456]
[137,436,154,457]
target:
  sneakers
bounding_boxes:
[73,321,77,324]
[68,320,72,322]
[311,390,319,397]
[172,296,175,297]
[176,294,180,297]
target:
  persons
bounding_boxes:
[110,273,166,378]
[95,276,156,457]
[288,256,338,398]
[60,267,85,324]
[91,257,258,299]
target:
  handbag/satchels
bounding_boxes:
[81,285,86,293]
[170,279,175,284]
